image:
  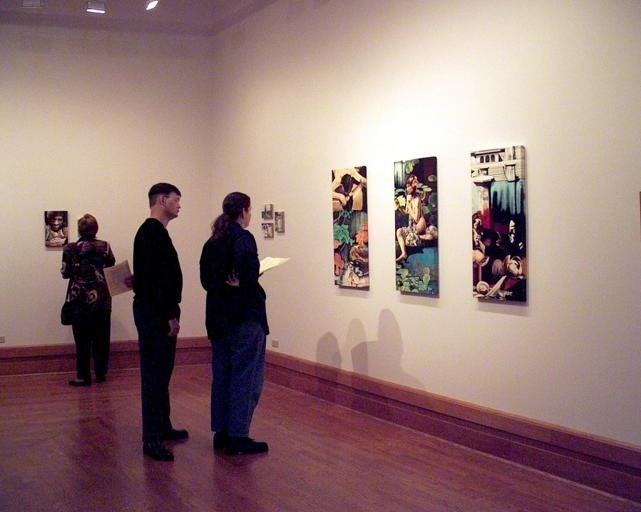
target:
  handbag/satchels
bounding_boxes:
[60,289,89,325]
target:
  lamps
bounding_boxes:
[19,0,160,15]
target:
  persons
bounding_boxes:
[45,211,68,247]
[200,191,270,455]
[124,182,189,461]
[60,213,116,386]
[394,175,433,261]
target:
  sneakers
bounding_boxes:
[69,379,91,386]
[213,433,269,456]
[162,429,188,440]
[143,441,174,461]
